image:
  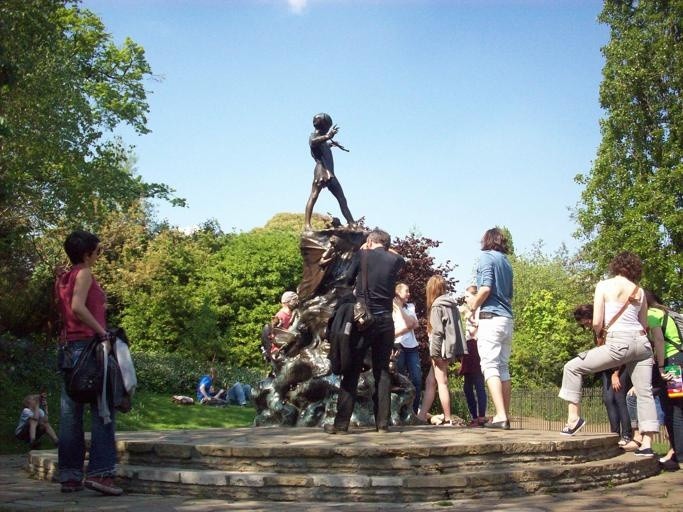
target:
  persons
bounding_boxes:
[392,282,421,415]
[210,382,252,408]
[269,291,298,353]
[574,305,632,445]
[557,250,660,457]
[456,286,487,427]
[324,231,406,436]
[622,385,674,463]
[15,394,60,449]
[304,113,355,232]
[643,288,683,469]
[197,368,217,403]
[418,275,469,427]
[466,228,513,430]
[54,230,123,494]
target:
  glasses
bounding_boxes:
[88,248,103,255]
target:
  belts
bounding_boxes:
[478,311,497,321]
[606,331,647,340]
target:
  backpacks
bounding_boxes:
[662,310,683,352]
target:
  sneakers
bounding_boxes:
[467,420,484,428]
[632,447,654,457]
[84,477,123,497]
[58,479,84,492]
[559,417,585,438]
[437,419,453,426]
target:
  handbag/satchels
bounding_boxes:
[63,336,107,405]
[347,249,375,334]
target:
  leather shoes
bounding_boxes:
[376,425,390,432]
[619,440,641,452]
[324,423,347,435]
[484,420,511,430]
